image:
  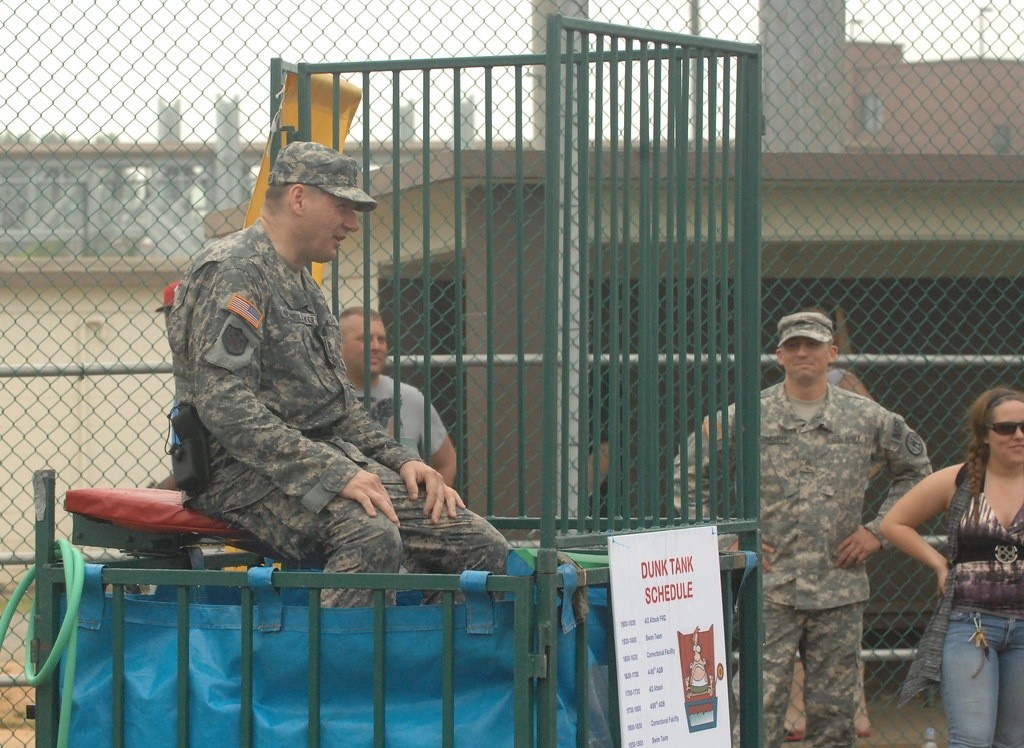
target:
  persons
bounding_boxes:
[165,140,509,609]
[779,303,874,741]
[147,281,183,492]
[586,431,609,533]
[338,306,457,488]
[672,312,932,748]
[877,385,1024,748]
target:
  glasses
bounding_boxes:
[985,421,1024,436]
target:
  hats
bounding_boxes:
[268,142,378,212]
[777,313,833,347]
